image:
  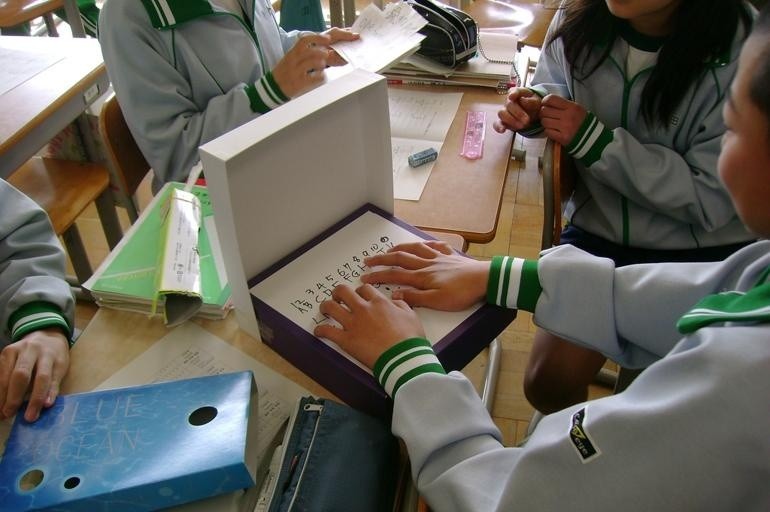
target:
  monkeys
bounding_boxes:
[408,147,438,168]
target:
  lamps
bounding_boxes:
[543,135,620,390]
[0,0,124,302]
[100,95,152,225]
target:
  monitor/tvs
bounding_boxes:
[81,182,233,321]
[382,32,531,89]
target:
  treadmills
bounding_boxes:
[461,111,486,160]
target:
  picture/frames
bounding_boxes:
[383,54,529,244]
[0,34,110,181]
[58,229,500,512]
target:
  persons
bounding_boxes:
[314,7,770,511]
[493,1,770,415]
[97,0,360,182]
[0,0,101,38]
[0,179,75,422]
